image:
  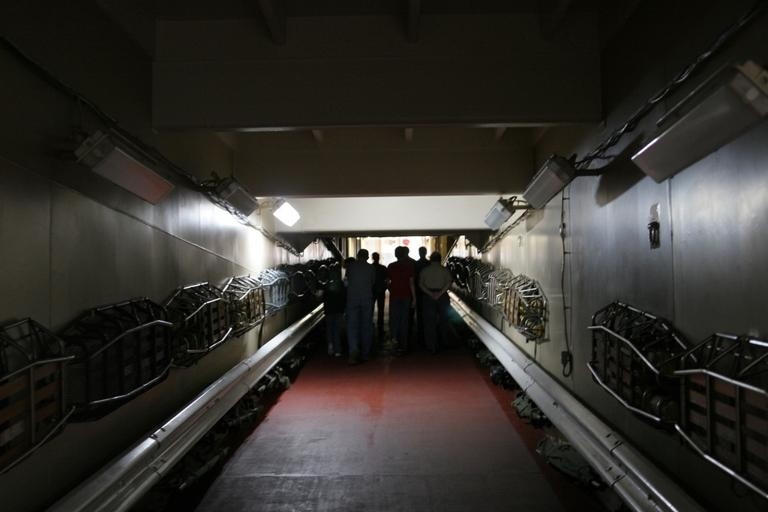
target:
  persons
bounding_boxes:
[323,244,455,365]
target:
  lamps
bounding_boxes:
[485,195,520,230]
[628,60,767,185]
[520,153,586,212]
[74,127,301,228]
